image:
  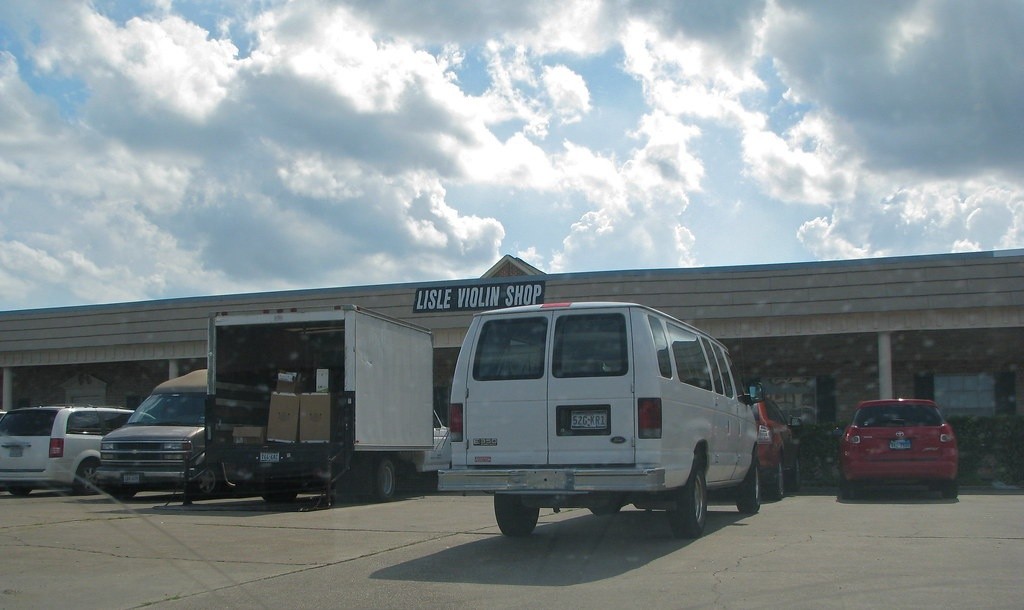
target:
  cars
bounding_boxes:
[747,394,804,500]
[833,397,959,500]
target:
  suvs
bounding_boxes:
[0,405,156,495]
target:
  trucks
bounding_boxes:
[95,369,220,500]
[204,304,452,501]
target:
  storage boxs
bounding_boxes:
[232,427,265,443]
[267,391,300,444]
[300,393,331,443]
[276,371,308,393]
[316,368,338,393]
[417,428,452,472]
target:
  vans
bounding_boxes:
[437,301,762,537]
